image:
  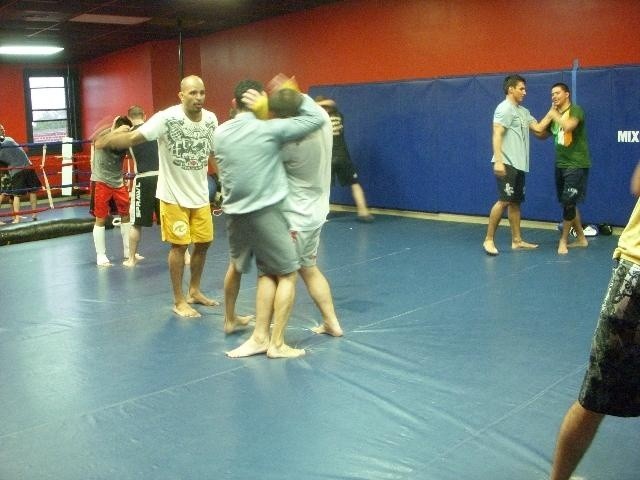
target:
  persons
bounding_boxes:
[484,76,551,254]
[1,125,42,224]
[535,83,591,253]
[223,72,343,362]
[118,106,194,269]
[91,74,222,319]
[209,78,327,361]
[89,115,143,268]
[315,93,379,223]
[552,160,639,479]
